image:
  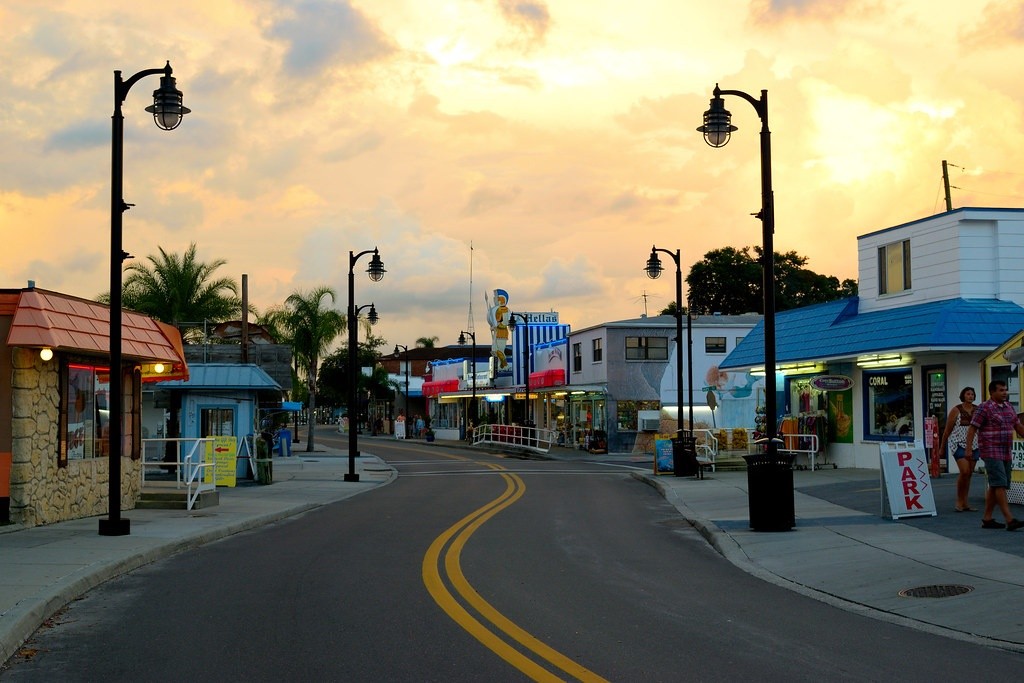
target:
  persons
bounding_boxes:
[965,378,1024,532]
[412,414,431,438]
[398,412,406,422]
[376,416,390,436]
[273,424,292,456]
[938,386,981,512]
[466,425,478,446]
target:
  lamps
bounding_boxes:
[39,347,53,361]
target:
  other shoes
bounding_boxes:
[955,506,970,512]
[1006,518,1024,531]
[981,520,1007,529]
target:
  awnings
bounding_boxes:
[422,380,460,398]
[529,369,565,390]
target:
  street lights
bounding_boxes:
[98,59,193,536]
[507,311,530,425]
[643,245,684,436]
[343,246,387,481]
[697,83,783,450]
[354,302,379,457]
[457,330,477,428]
[393,344,410,435]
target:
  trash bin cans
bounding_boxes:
[743,454,797,533]
[670,437,698,476]
